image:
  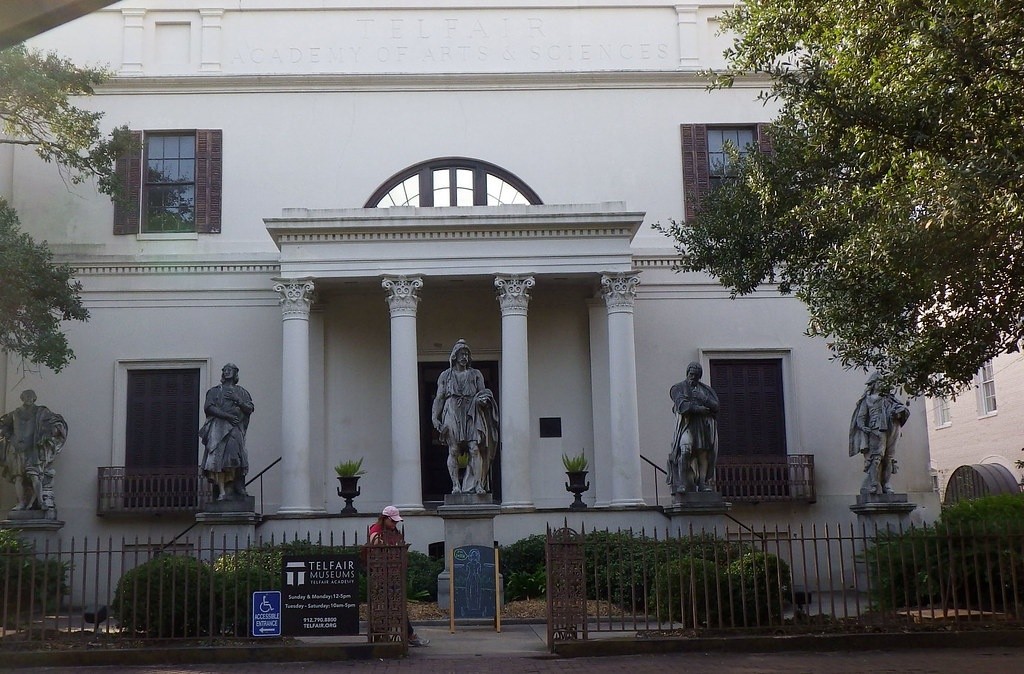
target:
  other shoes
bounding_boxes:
[410,633,430,645]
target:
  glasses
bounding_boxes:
[387,517,399,524]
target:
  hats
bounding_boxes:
[382,506,403,522]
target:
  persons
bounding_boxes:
[849,371,909,494]
[665,361,720,493]
[431,340,488,496]
[197,362,254,500]
[0,390,67,511]
[369,506,430,647]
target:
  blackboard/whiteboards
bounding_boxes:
[449,544,499,623]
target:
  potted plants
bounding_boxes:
[457,452,470,488]
[335,460,368,514]
[561,447,598,509]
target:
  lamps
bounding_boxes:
[790,590,812,617]
[84,602,107,635]
